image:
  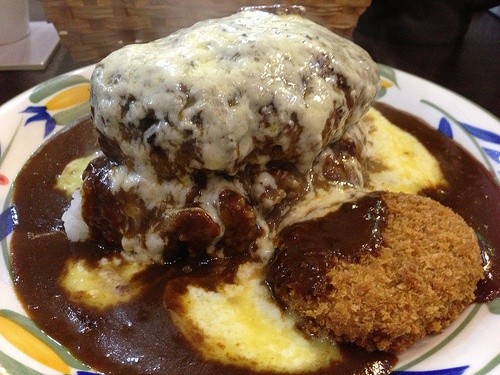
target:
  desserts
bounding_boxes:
[62,2,383,272]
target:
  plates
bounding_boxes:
[0,61,500,374]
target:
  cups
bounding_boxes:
[0,0,30,45]
